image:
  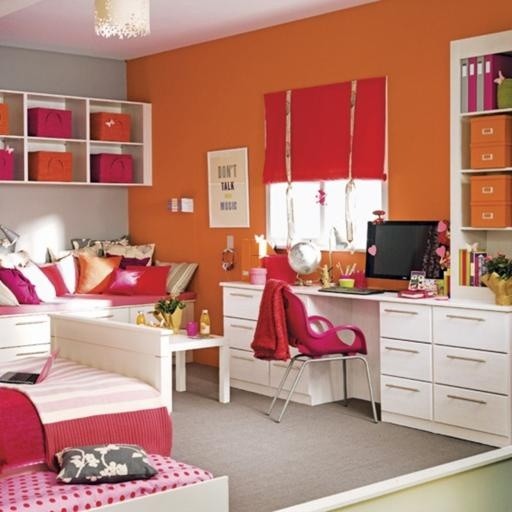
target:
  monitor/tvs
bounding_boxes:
[364,219,440,281]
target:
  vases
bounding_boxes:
[481,272,512,305]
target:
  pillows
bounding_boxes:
[1,239,199,305]
[55,443,158,485]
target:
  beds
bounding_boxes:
[1,291,197,364]
[1,312,173,467]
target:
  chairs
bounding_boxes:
[265,282,380,424]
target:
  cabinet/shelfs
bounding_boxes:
[223,287,311,396]
[449,28,512,304]
[1,300,194,376]
[0,89,153,187]
[378,300,510,439]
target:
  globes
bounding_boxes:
[288,241,321,286]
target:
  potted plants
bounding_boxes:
[154,296,186,331]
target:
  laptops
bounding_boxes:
[0,346,62,384]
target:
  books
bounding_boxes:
[398,288,434,299]
[458,247,490,288]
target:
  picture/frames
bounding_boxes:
[206,147,251,230]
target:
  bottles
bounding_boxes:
[199,308,211,337]
[135,311,145,325]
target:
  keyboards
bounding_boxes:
[318,285,383,296]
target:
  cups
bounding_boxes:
[352,271,363,288]
[338,278,355,287]
[249,267,267,285]
[497,79,512,109]
[186,321,198,337]
[341,274,351,278]
[435,279,444,296]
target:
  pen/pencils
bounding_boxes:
[338,261,357,276]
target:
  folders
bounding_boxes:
[461,55,512,113]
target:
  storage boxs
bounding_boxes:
[29,152,73,182]
[90,112,132,142]
[90,152,134,183]
[28,108,72,138]
[1,149,15,180]
[469,115,511,229]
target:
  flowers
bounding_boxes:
[481,252,511,278]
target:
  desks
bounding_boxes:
[172,331,231,404]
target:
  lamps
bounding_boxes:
[95,0,150,41]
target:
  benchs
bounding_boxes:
[1,458,230,512]
